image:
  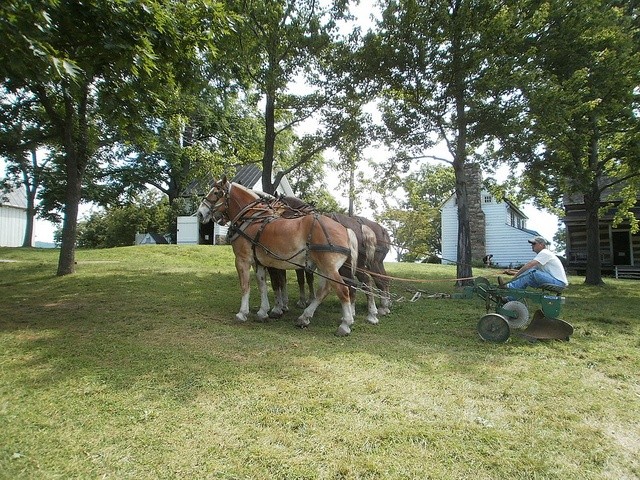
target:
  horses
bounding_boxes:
[273,191,392,314]
[196,175,358,337]
[237,181,382,325]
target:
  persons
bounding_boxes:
[483,254,493,268]
[498,238,568,291]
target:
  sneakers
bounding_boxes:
[498,276,506,289]
[502,296,509,304]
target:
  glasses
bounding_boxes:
[532,243,537,245]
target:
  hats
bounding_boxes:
[528,237,546,245]
